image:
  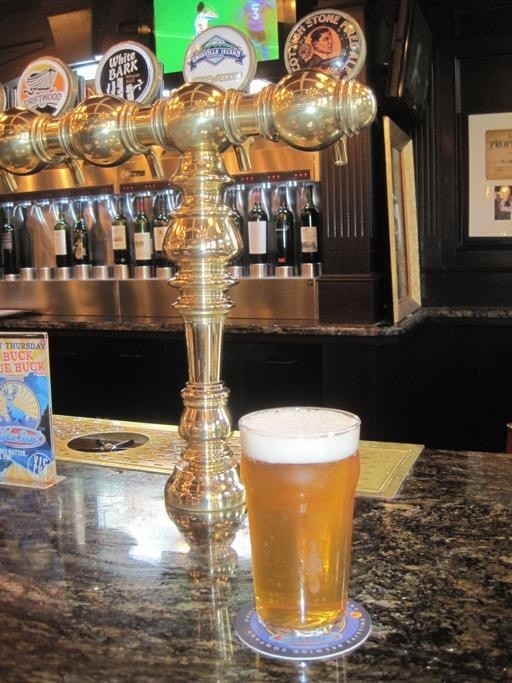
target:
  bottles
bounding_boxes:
[0,211,19,274]
[51,181,327,267]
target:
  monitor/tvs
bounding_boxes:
[386,0,436,124]
[134,0,297,90]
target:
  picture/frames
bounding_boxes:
[382,111,511,324]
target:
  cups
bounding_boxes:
[235,404,365,640]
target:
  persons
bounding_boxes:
[193,1,219,38]
[306,27,334,65]
[236,0,276,61]
